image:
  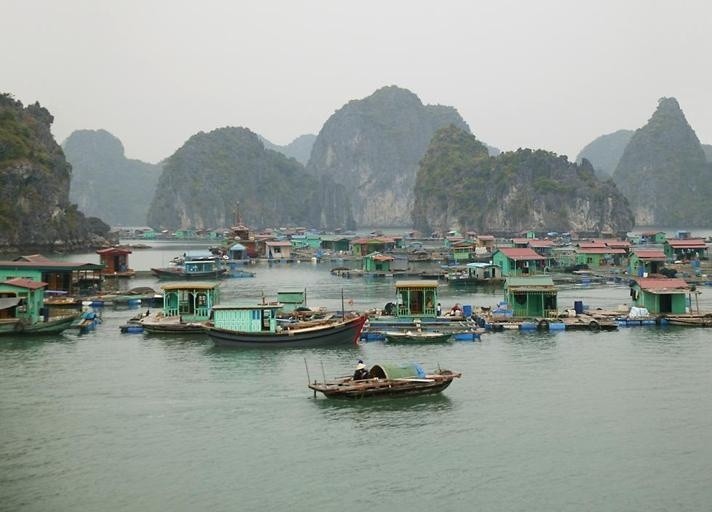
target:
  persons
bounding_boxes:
[437,303,441,315]
[451,303,460,312]
[427,299,432,306]
[353,360,368,380]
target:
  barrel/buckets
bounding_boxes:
[549,323,566,330]
[91,301,105,308]
[463,305,472,317]
[641,319,656,324]
[575,301,582,314]
[39,307,49,322]
[366,333,385,342]
[453,332,475,342]
[626,320,639,325]
[518,322,535,331]
[126,327,143,332]
[128,300,142,305]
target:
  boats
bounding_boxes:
[302,356,462,401]
